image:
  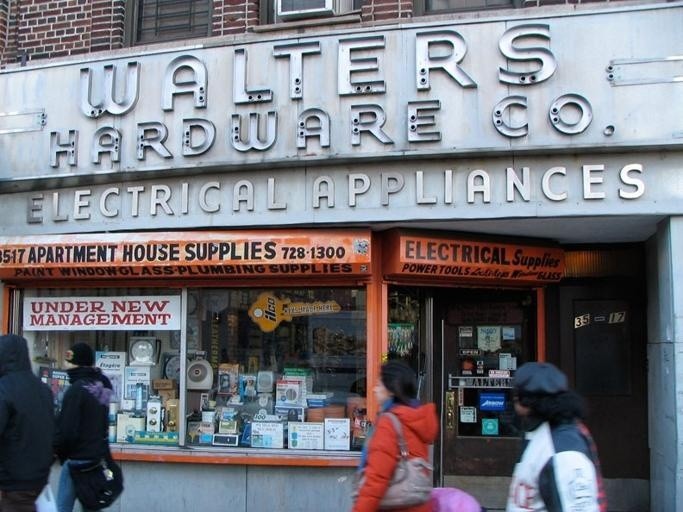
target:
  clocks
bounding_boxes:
[163,355,191,386]
[131,340,155,362]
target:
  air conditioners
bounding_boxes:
[277,0,334,22]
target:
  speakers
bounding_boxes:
[146,399,161,434]
[257,371,273,393]
[128,336,161,366]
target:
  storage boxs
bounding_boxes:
[95,351,178,445]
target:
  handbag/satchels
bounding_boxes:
[351,457,432,506]
[68,455,123,511]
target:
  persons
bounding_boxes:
[349,357,439,512]
[0,334,56,511]
[501,362,607,511]
[49,342,112,510]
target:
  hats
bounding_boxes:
[65,344,96,367]
[512,362,566,401]
